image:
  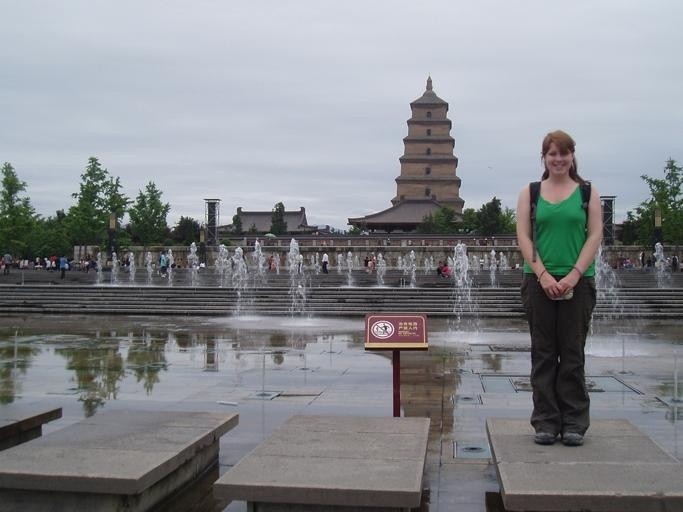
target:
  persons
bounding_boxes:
[1,251,513,280]
[514,129,604,448]
[619,251,680,274]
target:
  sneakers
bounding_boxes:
[561,431,585,446]
[534,432,559,445]
